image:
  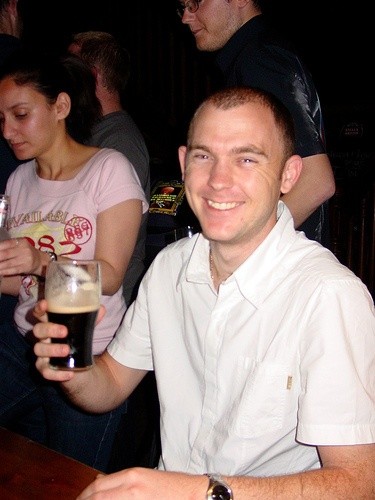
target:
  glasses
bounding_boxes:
[177,0,202,18]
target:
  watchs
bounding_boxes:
[204,473,233,500]
[45,251,58,262]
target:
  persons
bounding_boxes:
[0,1,149,452]
[176,1,336,247]
[0,69,149,470]
[30,83,374,500]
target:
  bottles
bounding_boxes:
[0,195,10,240]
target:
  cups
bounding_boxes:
[44,261,101,370]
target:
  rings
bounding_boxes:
[16,238,19,248]
